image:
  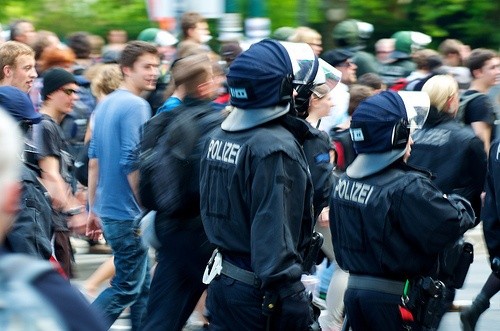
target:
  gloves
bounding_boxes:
[268,292,311,331]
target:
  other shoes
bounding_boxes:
[460,310,475,331]
[91,244,116,255]
[448,304,462,312]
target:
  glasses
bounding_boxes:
[59,89,75,95]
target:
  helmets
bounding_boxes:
[390,30,411,59]
[221,39,318,132]
[348,91,415,178]
[332,20,361,48]
[137,29,159,45]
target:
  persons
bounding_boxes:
[329,90,476,331]
[0,11,500,331]
[199,40,323,331]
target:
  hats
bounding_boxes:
[321,49,354,66]
[0,86,40,124]
[41,70,78,95]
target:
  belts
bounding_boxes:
[348,276,407,294]
[221,261,258,285]
